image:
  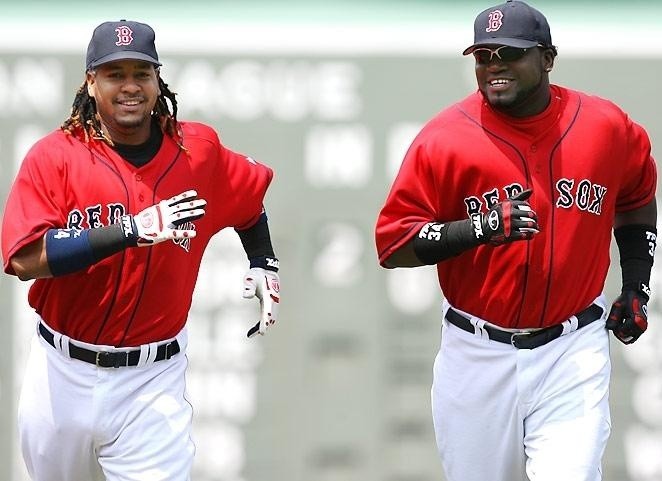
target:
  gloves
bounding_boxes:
[470,189,541,245]
[244,257,282,337]
[605,282,652,345]
[119,189,207,250]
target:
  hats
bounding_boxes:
[86,20,161,70]
[462,0,554,56]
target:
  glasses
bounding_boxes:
[472,46,544,64]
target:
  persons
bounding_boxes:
[0,19,282,481]
[375,0,658,481]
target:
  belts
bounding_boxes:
[38,322,180,368]
[444,304,607,350]
